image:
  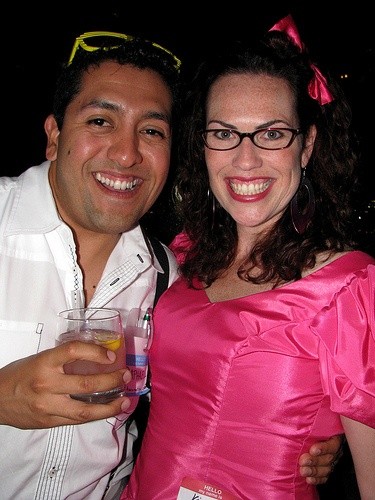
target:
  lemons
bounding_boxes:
[106,337,121,350]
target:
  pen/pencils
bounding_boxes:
[143,310,150,329]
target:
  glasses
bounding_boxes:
[66,31,181,75]
[197,125,306,152]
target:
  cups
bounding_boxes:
[54,307,127,404]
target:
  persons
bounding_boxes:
[0,32,345,500]
[121,28,374,500]
